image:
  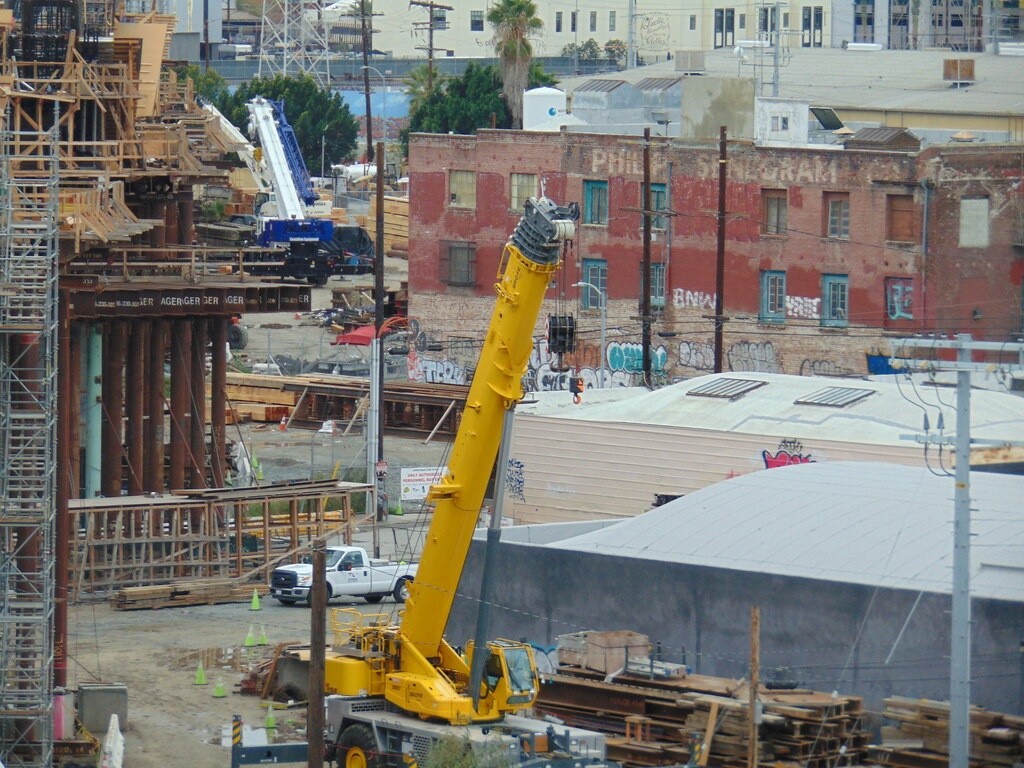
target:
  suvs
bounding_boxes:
[268,545,418,603]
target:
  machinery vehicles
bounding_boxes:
[321,188,616,768]
[189,95,335,279]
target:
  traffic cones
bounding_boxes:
[195,587,284,732]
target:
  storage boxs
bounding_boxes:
[585,631,649,674]
[558,630,592,666]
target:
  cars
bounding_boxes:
[332,217,377,275]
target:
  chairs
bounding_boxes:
[354,554,363,568]
